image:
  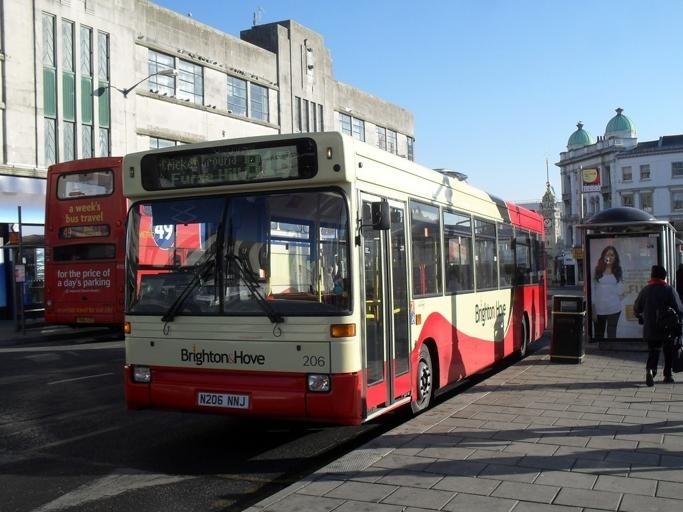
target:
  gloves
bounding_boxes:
[639,318,642,324]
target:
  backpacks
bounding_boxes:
[655,302,680,338]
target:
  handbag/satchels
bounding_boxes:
[672,344,682,373]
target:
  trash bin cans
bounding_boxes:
[550,295,587,364]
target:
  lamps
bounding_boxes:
[124,68,179,96]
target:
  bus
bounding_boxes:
[42,155,339,338]
[125,131,548,426]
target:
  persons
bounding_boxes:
[591,245,625,338]
[676,263,683,303]
[560,264,566,288]
[633,265,683,387]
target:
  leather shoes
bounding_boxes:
[645,368,653,387]
[663,376,674,383]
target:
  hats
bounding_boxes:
[651,265,666,280]
